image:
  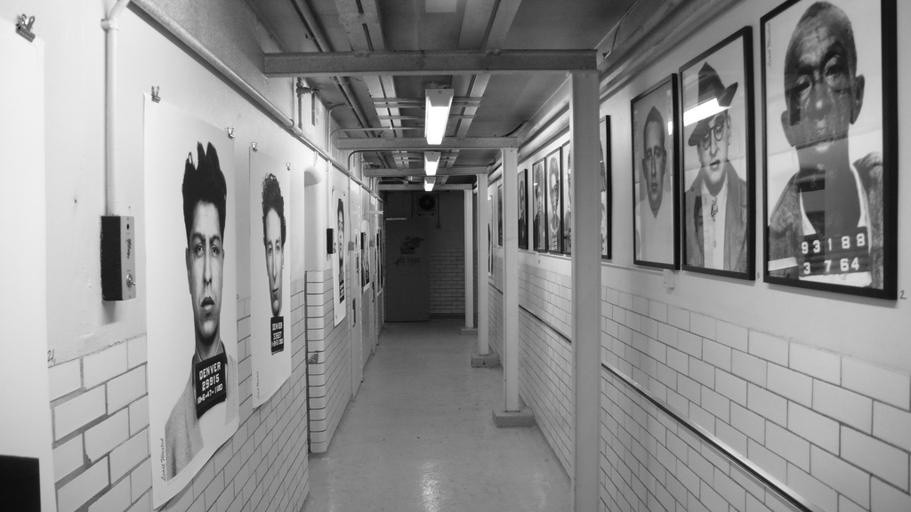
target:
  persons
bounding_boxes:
[684,61,749,273]
[515,139,613,258]
[635,103,679,266]
[335,198,347,307]
[261,172,288,352]
[761,0,886,290]
[163,141,249,482]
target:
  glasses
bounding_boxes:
[702,120,725,150]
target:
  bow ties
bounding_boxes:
[710,197,719,220]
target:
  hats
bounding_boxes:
[688,62,739,146]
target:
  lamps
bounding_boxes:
[422,85,455,194]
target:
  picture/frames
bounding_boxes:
[630,0,899,303]
[517,115,612,259]
